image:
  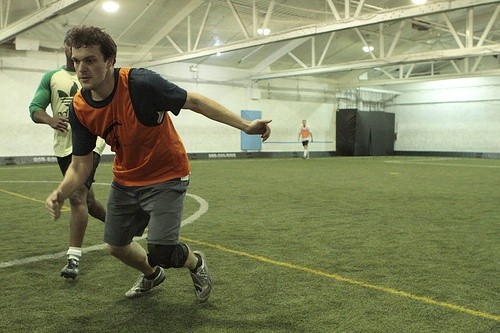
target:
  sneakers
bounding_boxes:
[126,265,166,298]
[59,258,80,280]
[189,250,212,303]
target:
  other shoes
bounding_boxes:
[301,156,306,159]
[306,156,309,159]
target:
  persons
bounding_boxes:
[29,28,107,279]
[45,24,273,302]
[298,119,313,159]
